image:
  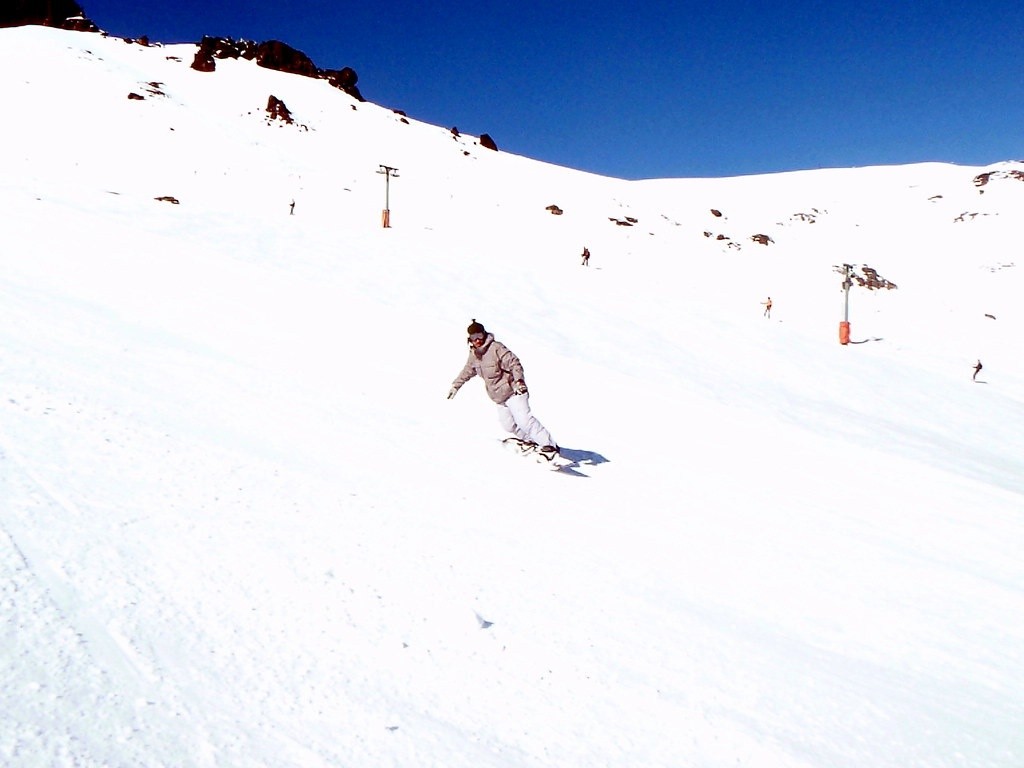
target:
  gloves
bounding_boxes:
[447,387,458,399]
[513,382,527,395]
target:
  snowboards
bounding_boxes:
[503,438,576,471]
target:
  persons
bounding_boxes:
[760,297,772,319]
[972,360,982,380]
[446,319,560,462]
[581,249,590,266]
[289,199,295,214]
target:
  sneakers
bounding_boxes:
[537,445,561,463]
[521,440,537,456]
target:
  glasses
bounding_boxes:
[470,333,484,341]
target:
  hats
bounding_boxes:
[467,319,485,333]
